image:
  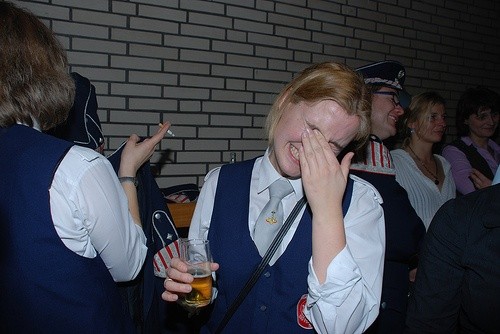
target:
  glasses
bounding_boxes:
[368,89,402,106]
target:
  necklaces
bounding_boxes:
[408,144,439,185]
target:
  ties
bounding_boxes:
[252,177,294,266]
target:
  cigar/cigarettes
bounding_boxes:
[159,122,176,136]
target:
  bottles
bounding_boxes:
[229,151,238,164]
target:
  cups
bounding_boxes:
[179,237,214,307]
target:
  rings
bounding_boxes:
[163,279,172,288]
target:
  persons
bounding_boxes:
[0,1,171,334]
[162,62,385,334]
[442,87,500,195]
[391,91,456,232]
[349,59,424,333]
[405,183,500,333]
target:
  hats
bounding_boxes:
[353,59,407,90]
[42,70,104,152]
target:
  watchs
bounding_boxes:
[119,177,139,186]
